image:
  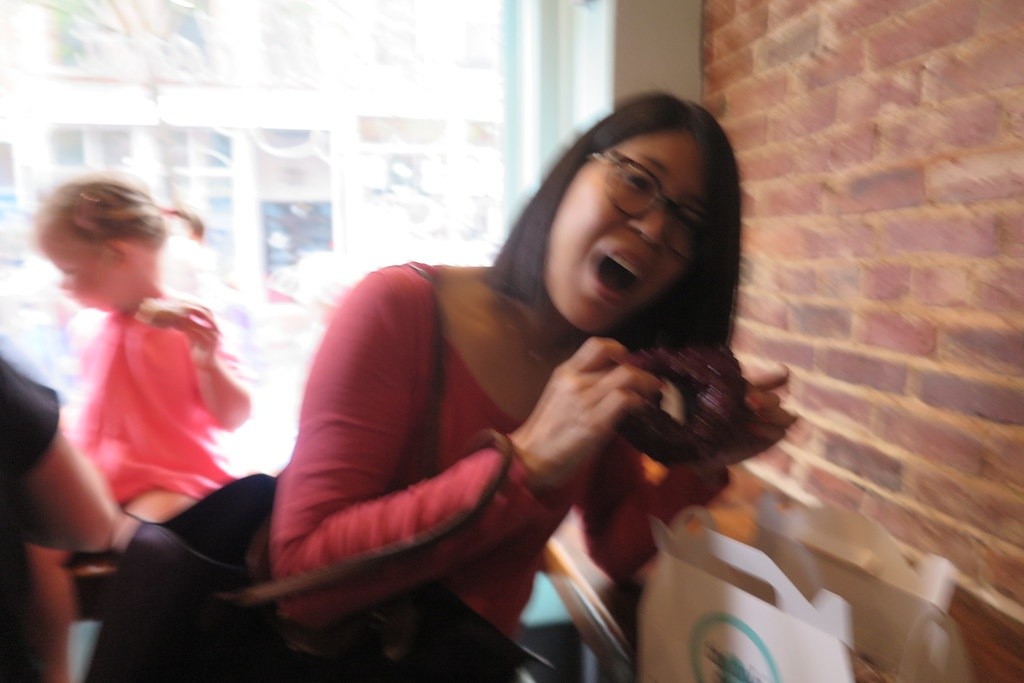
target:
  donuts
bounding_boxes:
[614,339,745,464]
[133,297,188,326]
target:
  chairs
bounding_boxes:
[514,537,641,683]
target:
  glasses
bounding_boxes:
[586,150,711,264]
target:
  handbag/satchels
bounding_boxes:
[85,270,448,683]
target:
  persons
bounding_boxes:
[271,91,797,682]
[36,174,251,575]
[0,357,113,683]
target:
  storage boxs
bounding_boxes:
[638,490,973,683]
